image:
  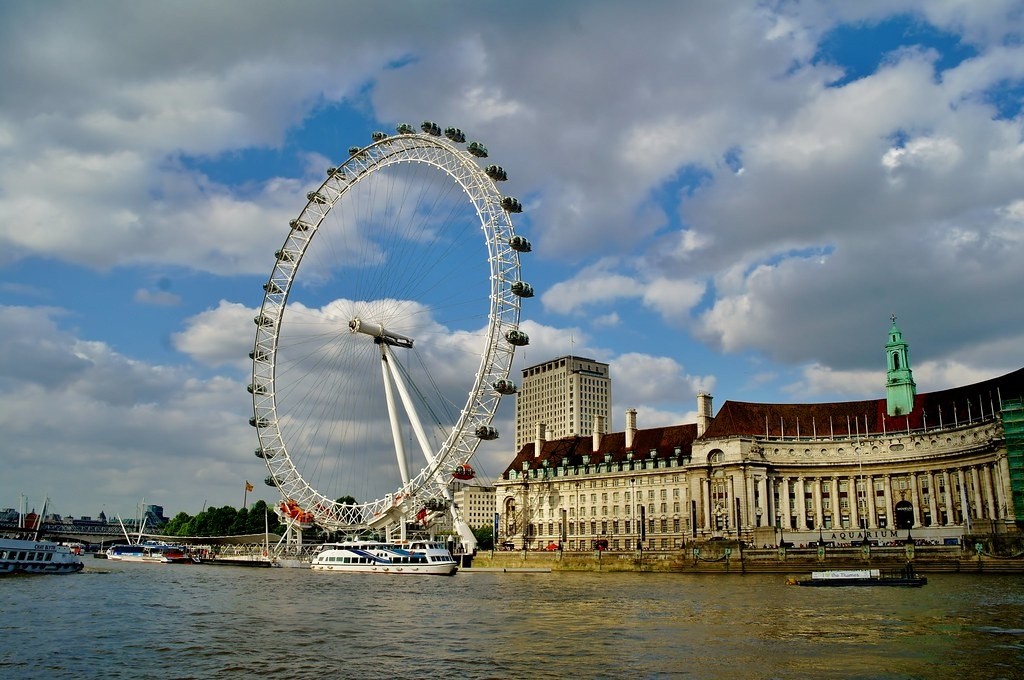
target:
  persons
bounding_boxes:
[494,547,665,551]
[748,538,940,550]
[172,542,216,560]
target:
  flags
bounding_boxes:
[246,481,254,492]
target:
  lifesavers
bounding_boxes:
[409,550,415,554]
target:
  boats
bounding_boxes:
[107,542,191,564]
[786,561,929,588]
[0,523,84,575]
[309,541,459,575]
